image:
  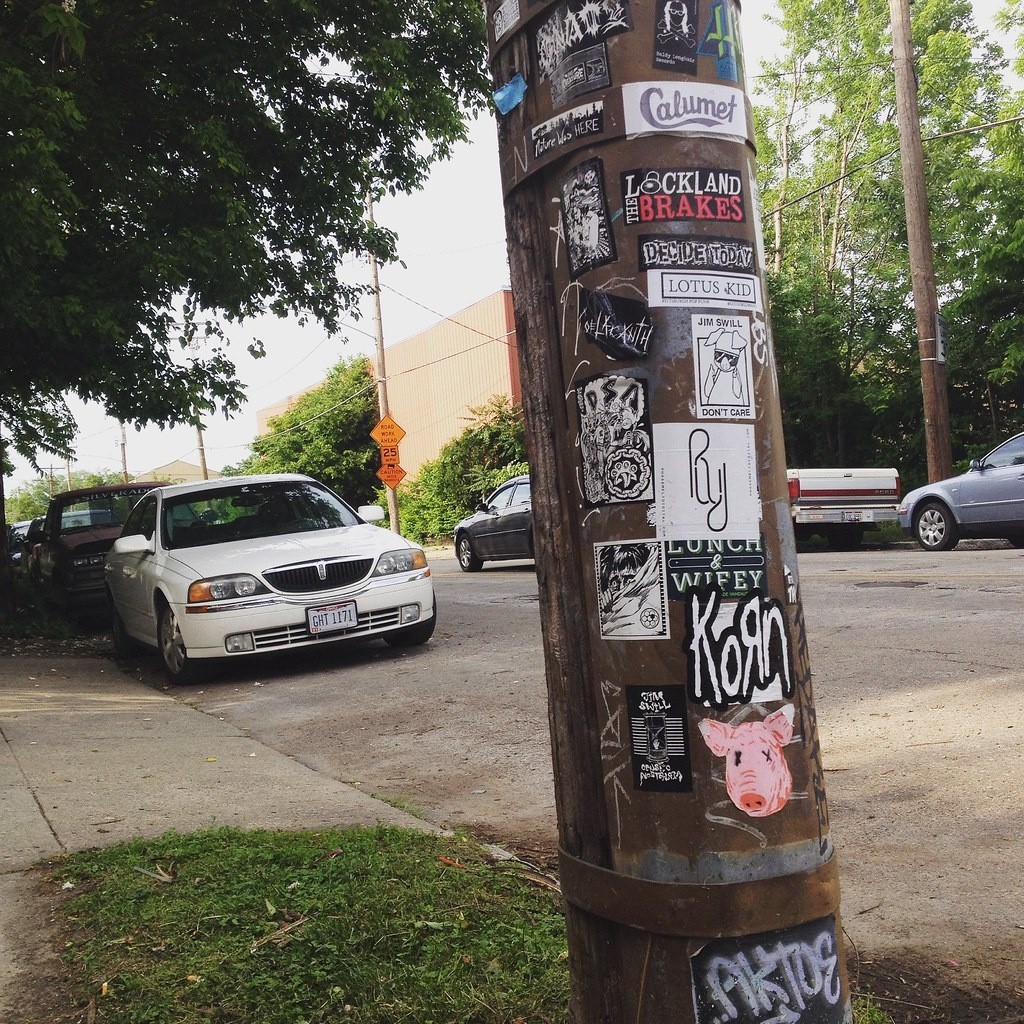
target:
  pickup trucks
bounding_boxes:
[787,468,900,548]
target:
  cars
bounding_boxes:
[454,475,535,573]
[899,432,1023,550]
[0,473,436,686]
